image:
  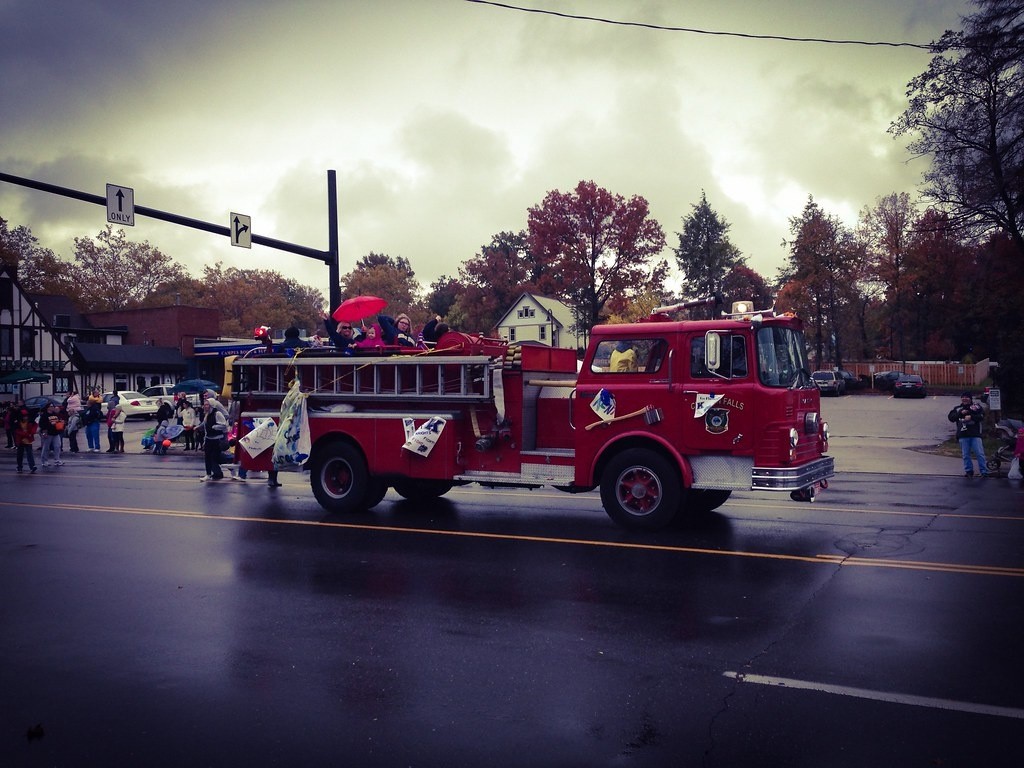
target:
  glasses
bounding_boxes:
[342,326,352,330]
[50,406,54,408]
[399,321,410,327]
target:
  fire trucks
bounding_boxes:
[231,310,836,535]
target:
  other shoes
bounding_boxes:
[981,473,989,477]
[965,470,974,477]
[268,481,282,486]
[15,467,22,473]
[232,476,248,482]
[30,466,37,474]
[206,476,221,481]
[200,475,212,481]
[5,444,13,448]
[37,434,205,454]
[11,445,17,450]
[42,461,52,467]
[54,460,66,466]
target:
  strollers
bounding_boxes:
[985,418,1024,471]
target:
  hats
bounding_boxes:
[285,326,299,338]
[115,404,122,410]
[960,391,973,400]
[21,410,28,414]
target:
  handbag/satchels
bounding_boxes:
[1008,457,1023,479]
[80,404,103,426]
[269,378,312,467]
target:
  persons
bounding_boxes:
[318,309,449,355]
[1014,428,1024,475]
[4,387,126,472]
[948,391,988,478]
[153,388,282,486]
[278,327,310,354]
[610,340,638,373]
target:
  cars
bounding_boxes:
[99,390,159,421]
[810,370,905,397]
[894,374,927,399]
[2,394,91,429]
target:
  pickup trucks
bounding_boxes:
[142,384,202,418]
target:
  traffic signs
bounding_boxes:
[106,183,134,226]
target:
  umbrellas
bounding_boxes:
[171,379,220,409]
[331,295,387,327]
